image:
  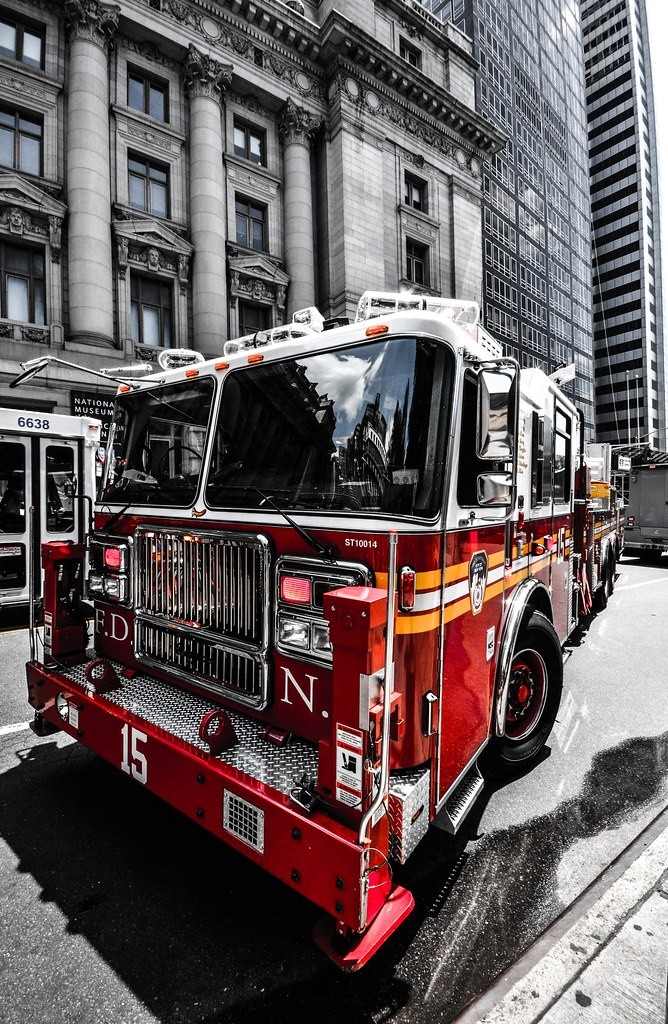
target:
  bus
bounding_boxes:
[0,408,105,609]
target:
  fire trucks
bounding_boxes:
[11,292,626,973]
